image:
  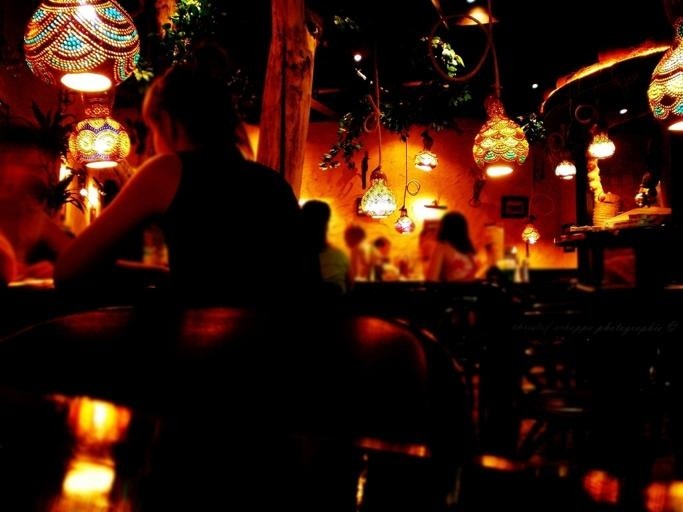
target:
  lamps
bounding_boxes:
[521,196,539,242]
[66,93,131,170]
[394,136,421,236]
[361,46,398,219]
[21,0,144,99]
[555,141,576,181]
[648,0,683,131]
[472,1,528,177]
[414,121,438,171]
[588,119,615,159]
[423,201,447,219]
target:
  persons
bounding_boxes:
[54,62,324,400]
[428,213,477,282]
[302,202,353,295]
[0,142,70,283]
